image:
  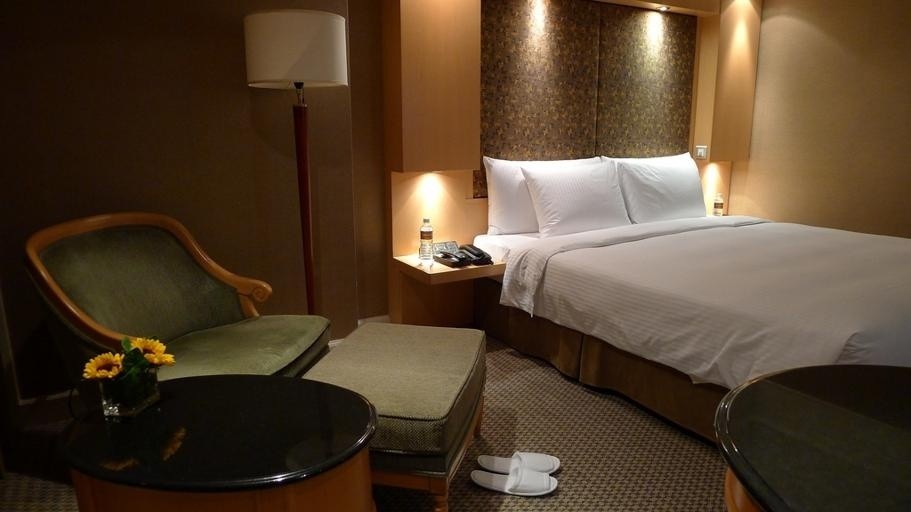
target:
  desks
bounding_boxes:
[713,359,911,506]
[72,376,374,510]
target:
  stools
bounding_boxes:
[303,323,485,508]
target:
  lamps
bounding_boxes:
[243,9,350,313]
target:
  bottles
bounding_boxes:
[420,218,433,266]
[713,193,724,216]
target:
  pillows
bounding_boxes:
[616,152,709,221]
[519,161,630,233]
[482,156,600,233]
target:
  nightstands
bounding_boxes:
[387,167,508,324]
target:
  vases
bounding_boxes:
[101,379,160,421]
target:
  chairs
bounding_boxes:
[26,211,332,404]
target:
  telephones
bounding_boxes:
[458,244,493,266]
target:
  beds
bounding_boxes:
[474,211,910,436]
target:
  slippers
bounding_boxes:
[470,467,558,495]
[477,453,561,473]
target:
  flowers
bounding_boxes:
[79,335,171,378]
